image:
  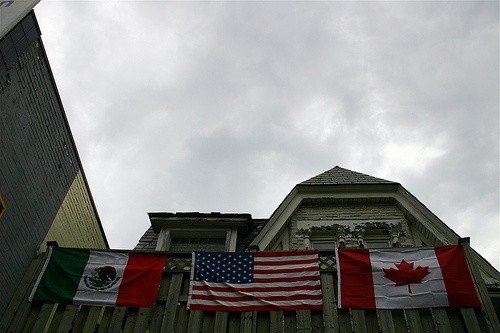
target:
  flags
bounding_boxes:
[336,243,482,310]
[26,245,169,308]
[186,250,323,311]
